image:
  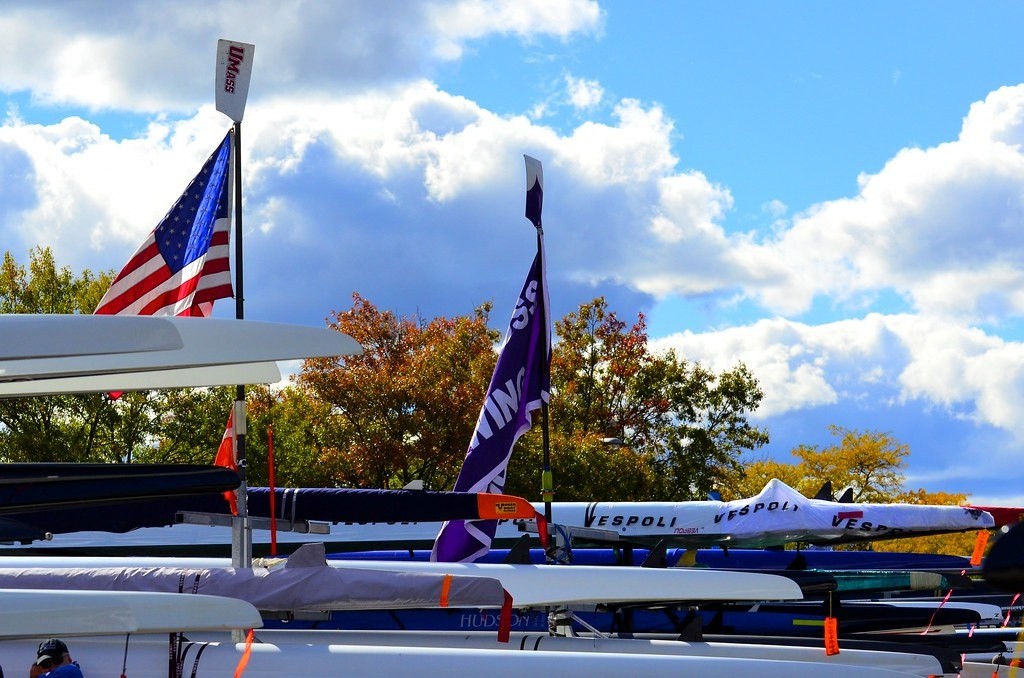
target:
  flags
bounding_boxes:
[426,229,552,563]
[90,125,234,399]
[213,401,248,515]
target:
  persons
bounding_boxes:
[29,639,84,678]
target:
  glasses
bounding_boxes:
[41,654,67,668]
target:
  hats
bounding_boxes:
[37,639,69,665]
